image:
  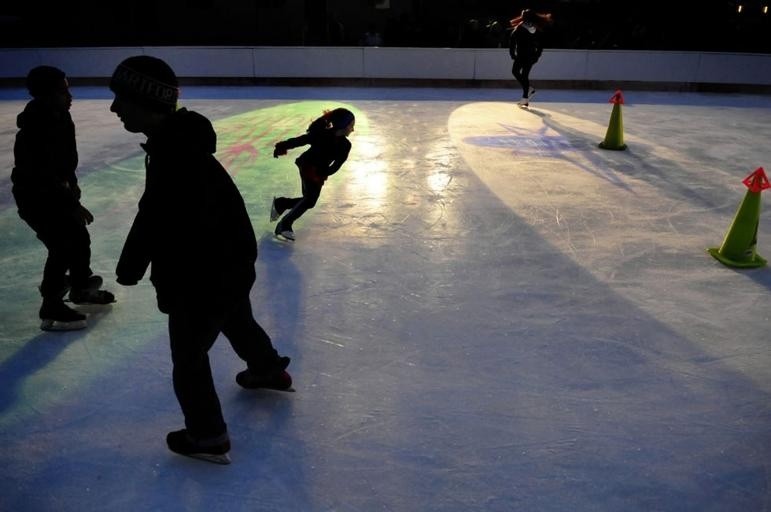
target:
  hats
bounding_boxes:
[109,55,179,114]
[26,67,65,99]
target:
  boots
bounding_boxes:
[40,278,86,322]
[71,278,115,302]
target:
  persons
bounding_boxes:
[507,8,554,107]
[270,106,356,242]
[448,18,488,48]
[8,63,116,323]
[108,54,293,456]
[481,18,506,48]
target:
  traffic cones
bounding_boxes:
[599,89,628,151]
[707,168,771,268]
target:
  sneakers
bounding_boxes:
[235,356,294,390]
[520,85,535,106]
[272,198,284,220]
[276,224,297,240]
[166,426,230,456]
